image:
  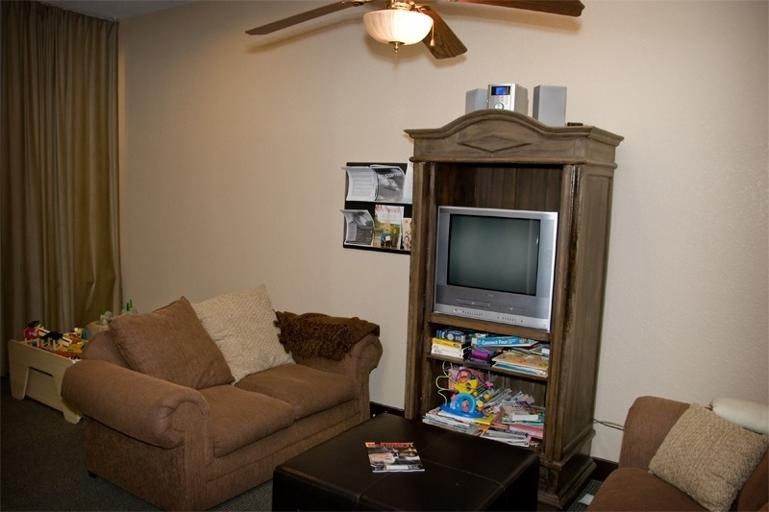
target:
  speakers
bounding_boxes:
[466,88,487,114]
[534,85,567,127]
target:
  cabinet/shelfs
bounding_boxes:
[404,107,625,512]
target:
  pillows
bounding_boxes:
[645,402,769,512]
[190,282,297,388]
[107,294,236,391]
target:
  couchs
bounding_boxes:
[58,311,385,512]
[588,395,769,512]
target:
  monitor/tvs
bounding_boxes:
[433,206,560,333]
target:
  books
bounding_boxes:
[430,335,548,379]
[342,164,412,251]
[365,442,425,474]
[422,388,544,448]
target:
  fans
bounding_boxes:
[243,0,584,59]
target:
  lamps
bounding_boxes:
[361,1,436,56]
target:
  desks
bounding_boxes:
[7,338,88,426]
